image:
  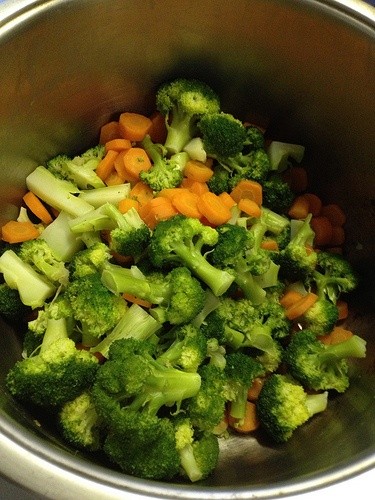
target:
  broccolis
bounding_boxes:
[0,68,366,482]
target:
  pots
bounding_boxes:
[0,0,375,500]
